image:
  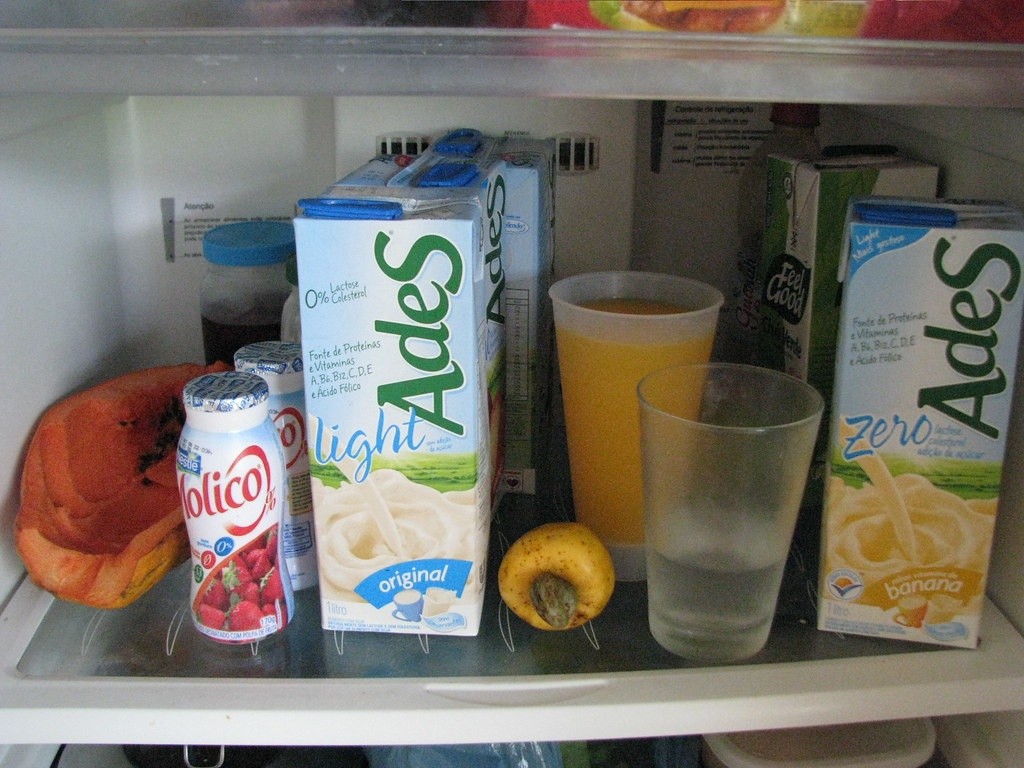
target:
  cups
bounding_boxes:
[549,271,726,580]
[635,362,825,663]
